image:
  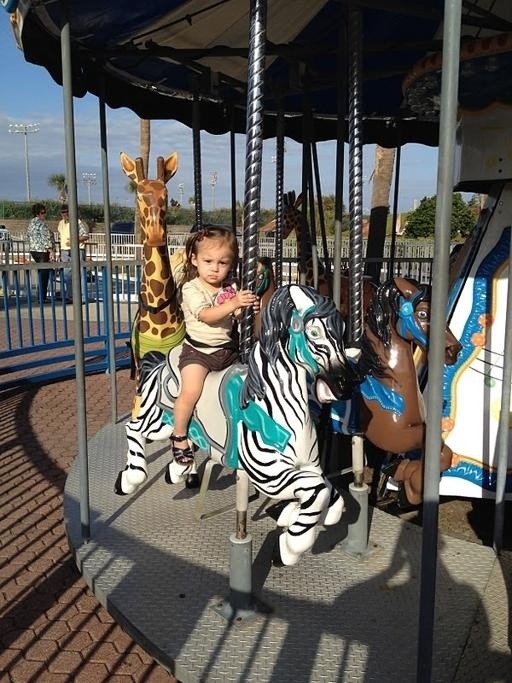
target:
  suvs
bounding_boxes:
[111,221,134,252]
[190,222,241,236]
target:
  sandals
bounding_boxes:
[169,433,194,465]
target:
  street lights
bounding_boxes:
[82,170,97,205]
[272,155,277,163]
[208,169,217,210]
[177,182,184,203]
[7,120,41,203]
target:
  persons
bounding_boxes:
[56,202,91,302]
[27,200,56,303]
[165,222,261,467]
[1,223,14,253]
